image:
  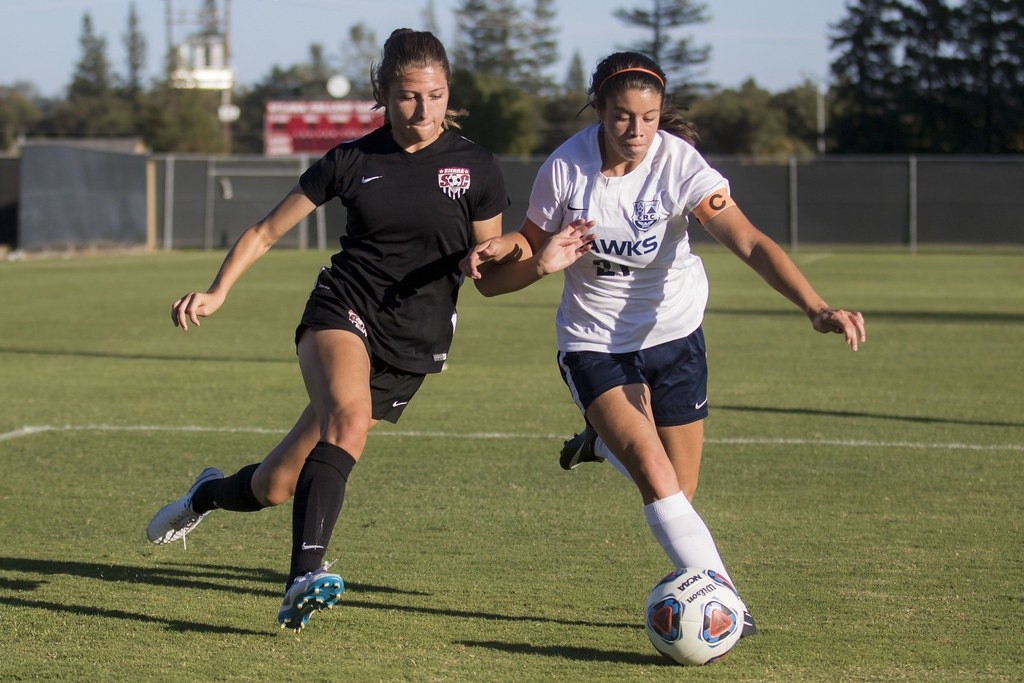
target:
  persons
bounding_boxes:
[458,49,868,636]
[145,26,597,635]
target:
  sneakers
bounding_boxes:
[147,468,224,550]
[278,559,344,634]
[559,423,607,470]
[737,594,756,636]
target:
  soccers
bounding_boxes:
[646,569,745,666]
